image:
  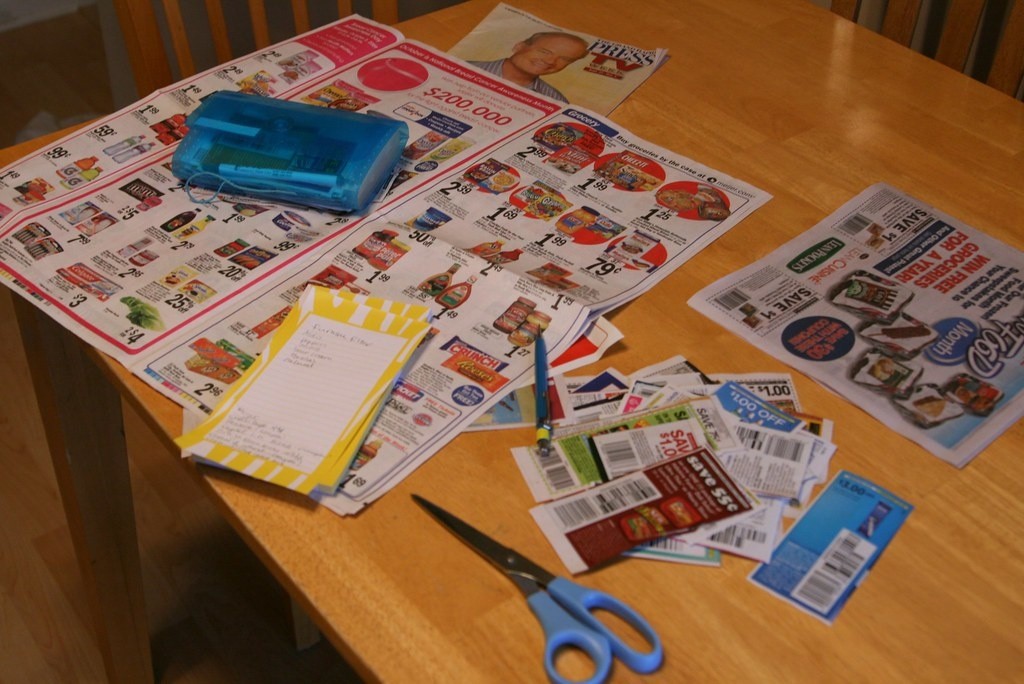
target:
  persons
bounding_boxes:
[466,32,589,105]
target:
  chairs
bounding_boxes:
[113,0,398,99]
[826,0,1024,98]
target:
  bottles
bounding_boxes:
[489,248,524,266]
[555,205,600,238]
[158,207,202,232]
[149,112,190,135]
[155,126,190,146]
[416,263,462,297]
[493,296,552,348]
[55,155,99,180]
[352,229,411,271]
[112,141,156,164]
[471,239,505,257]
[434,275,477,309]
[59,166,104,190]
[172,214,216,241]
[103,134,146,156]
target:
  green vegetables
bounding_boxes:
[120,296,164,331]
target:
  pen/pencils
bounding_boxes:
[201,160,339,188]
[533,323,553,456]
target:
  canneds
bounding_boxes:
[401,130,476,165]
[493,296,551,348]
[367,239,410,271]
[129,250,160,267]
[13,191,45,207]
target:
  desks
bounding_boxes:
[0,0,1024,684]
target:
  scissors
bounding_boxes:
[410,491,665,684]
